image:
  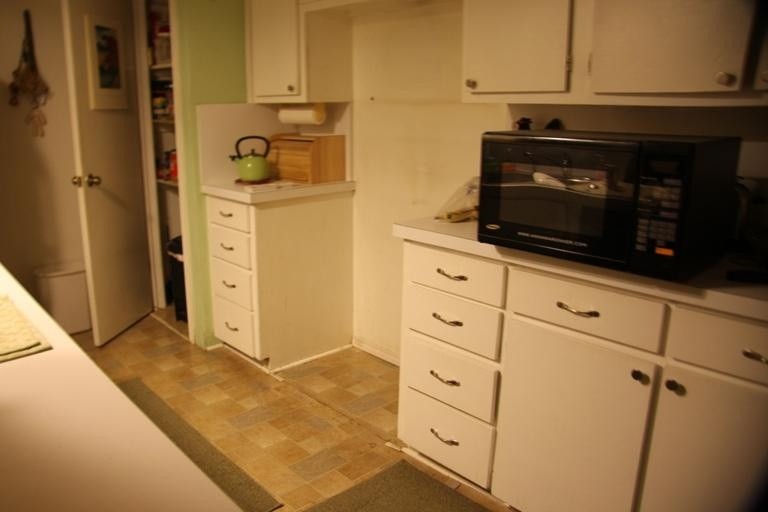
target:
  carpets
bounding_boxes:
[301,458,492,512]
[115,376,284,512]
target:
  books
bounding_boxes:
[0,295,39,358]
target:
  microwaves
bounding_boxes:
[478,128,741,288]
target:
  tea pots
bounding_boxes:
[228,135,271,184]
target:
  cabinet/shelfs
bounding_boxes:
[199,182,356,375]
[244,0,354,104]
[634,292,767,512]
[460,0,580,105]
[60,1,197,349]
[580,1,768,107]
[394,237,510,502]
[488,264,670,512]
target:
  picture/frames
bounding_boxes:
[84,13,129,112]
